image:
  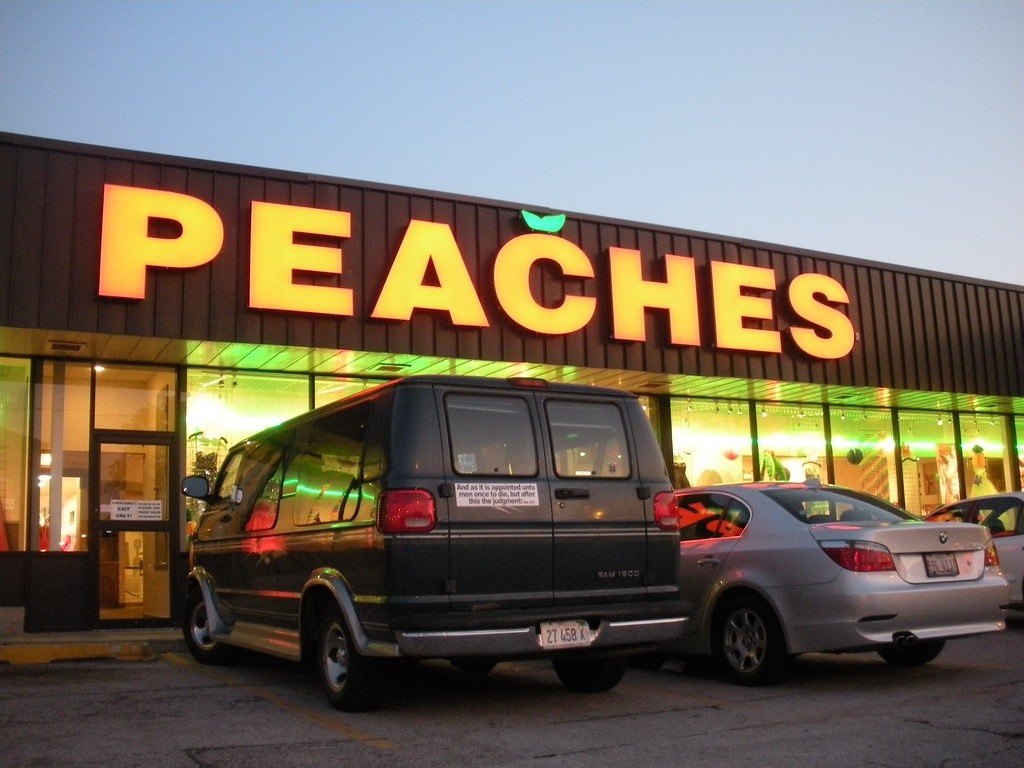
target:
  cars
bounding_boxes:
[662,478,1015,688]
[921,491,1024,619]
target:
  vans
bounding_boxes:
[173,375,695,709]
